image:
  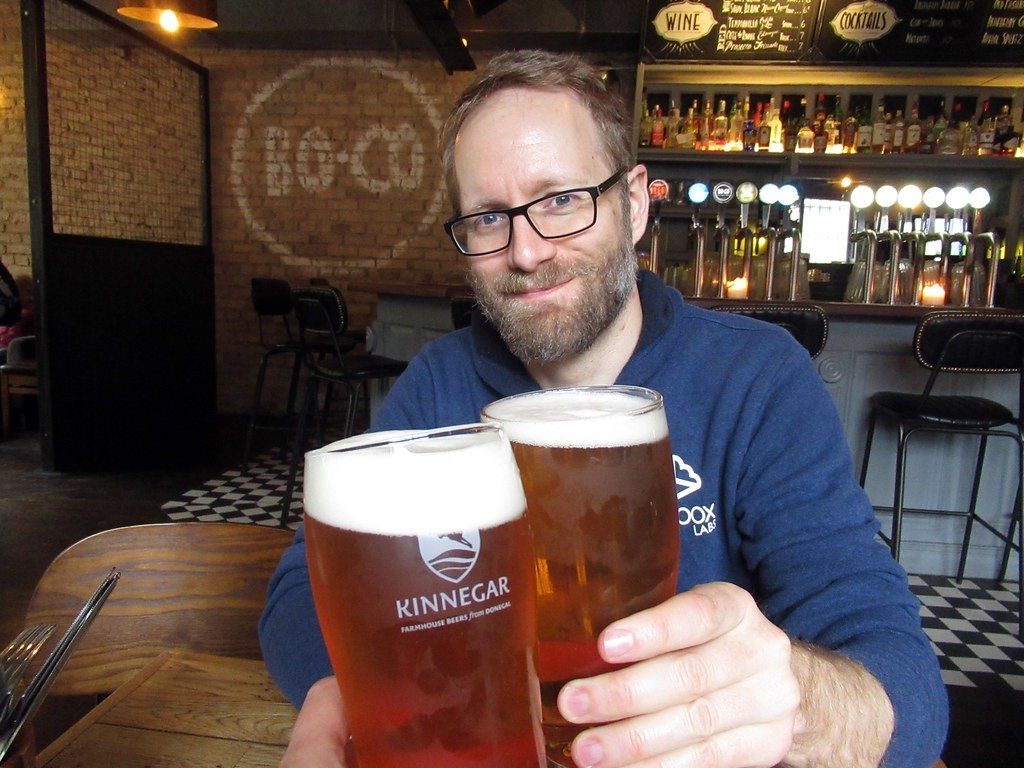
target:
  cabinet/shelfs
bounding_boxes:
[628,61,1024,318]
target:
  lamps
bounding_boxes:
[116,1,217,30]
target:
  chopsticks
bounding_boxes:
[0,567,121,765]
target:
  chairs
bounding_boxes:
[857,310,1024,643]
[22,522,301,751]
[244,276,407,525]
[712,301,829,361]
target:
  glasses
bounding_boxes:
[444,165,629,257]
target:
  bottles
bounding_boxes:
[637,93,1020,157]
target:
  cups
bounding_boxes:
[304,423,548,768]
[480,383,680,768]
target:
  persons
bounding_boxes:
[257,51,950,768]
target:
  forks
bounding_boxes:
[0,624,56,729]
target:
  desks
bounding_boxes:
[13,648,303,768]
